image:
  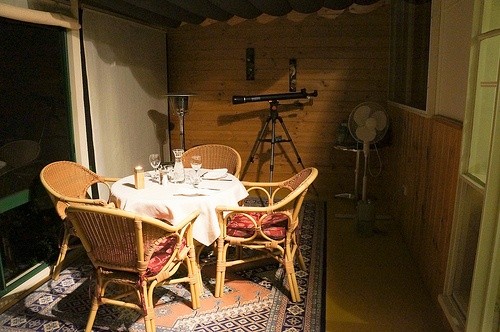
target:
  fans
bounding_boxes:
[335,101,392,222]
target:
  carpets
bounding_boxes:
[0,197,327,332]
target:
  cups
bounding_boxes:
[190,156,203,172]
[190,173,202,187]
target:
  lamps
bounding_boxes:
[161,92,197,151]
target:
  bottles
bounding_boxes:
[173,149,185,183]
[134,167,144,189]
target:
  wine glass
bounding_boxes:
[149,154,161,173]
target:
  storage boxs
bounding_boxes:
[134,168,145,189]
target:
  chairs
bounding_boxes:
[214,167,318,303]
[65,205,201,332]
[181,144,240,182]
[39,160,122,281]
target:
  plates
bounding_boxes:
[201,169,227,179]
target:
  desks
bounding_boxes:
[333,145,381,200]
[109,169,250,268]
